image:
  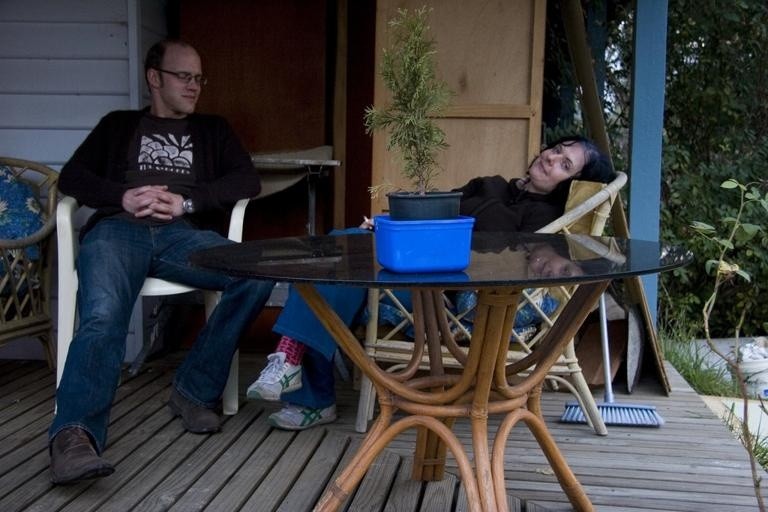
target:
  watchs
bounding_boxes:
[182,194,196,216]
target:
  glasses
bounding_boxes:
[152,66,207,85]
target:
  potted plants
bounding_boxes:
[362,4,474,273]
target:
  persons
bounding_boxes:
[48,40,277,483]
[246,136,613,431]
[509,235,597,278]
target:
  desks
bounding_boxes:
[186,232,694,512]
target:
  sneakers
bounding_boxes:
[247,352,302,401]
[269,404,337,430]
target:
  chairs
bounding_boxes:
[354,171,627,436]
[53,196,249,417]
[0,156,59,374]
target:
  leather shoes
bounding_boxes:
[168,389,220,432]
[50,427,115,484]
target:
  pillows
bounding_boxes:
[563,180,609,232]
[457,287,560,327]
[565,234,611,261]
[0,165,49,297]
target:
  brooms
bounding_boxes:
[557,290,665,427]
